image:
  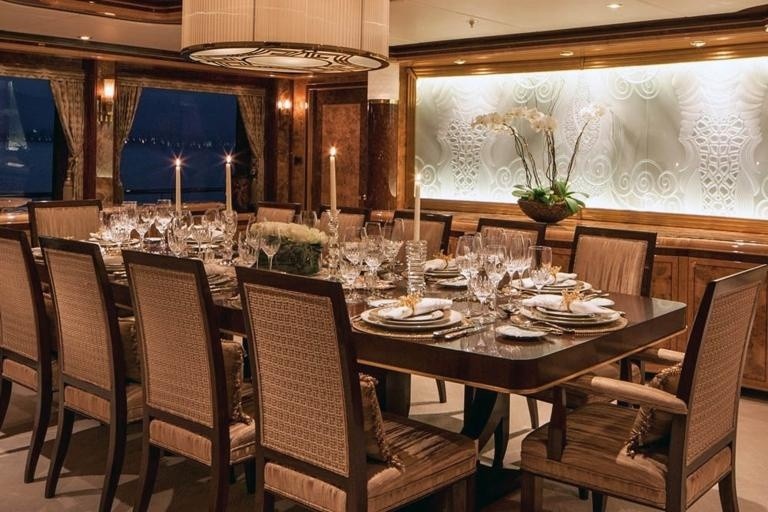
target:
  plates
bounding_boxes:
[495,324,549,339]
[206,272,236,292]
[520,295,621,326]
[508,273,592,297]
[437,279,467,287]
[424,266,459,277]
[105,263,128,279]
[361,304,462,330]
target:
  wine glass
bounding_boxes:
[298,210,318,227]
[338,217,405,303]
[455,226,552,316]
[239,217,281,270]
[97,199,238,253]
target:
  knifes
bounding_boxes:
[434,321,495,339]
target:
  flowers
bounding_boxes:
[472,80,605,213]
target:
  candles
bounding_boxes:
[175,158,181,211]
[413,175,420,240]
[225,155,233,210]
[329,147,337,211]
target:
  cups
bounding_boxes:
[406,240,427,297]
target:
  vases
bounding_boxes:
[518,200,577,223]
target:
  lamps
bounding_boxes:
[277,98,292,129]
[366,61,400,106]
[97,79,115,130]
[180,0,390,75]
